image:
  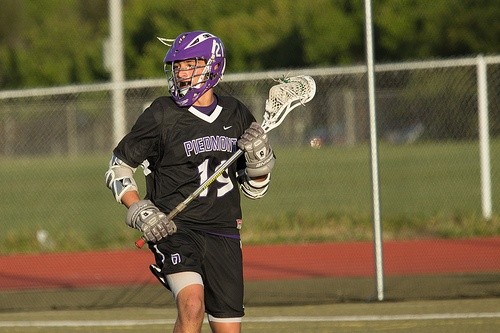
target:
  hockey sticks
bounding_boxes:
[134,75,316,250]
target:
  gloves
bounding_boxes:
[238,122,276,177]
[125,200,177,243]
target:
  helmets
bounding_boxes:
[163,30,226,106]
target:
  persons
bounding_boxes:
[104,31,274,333]
